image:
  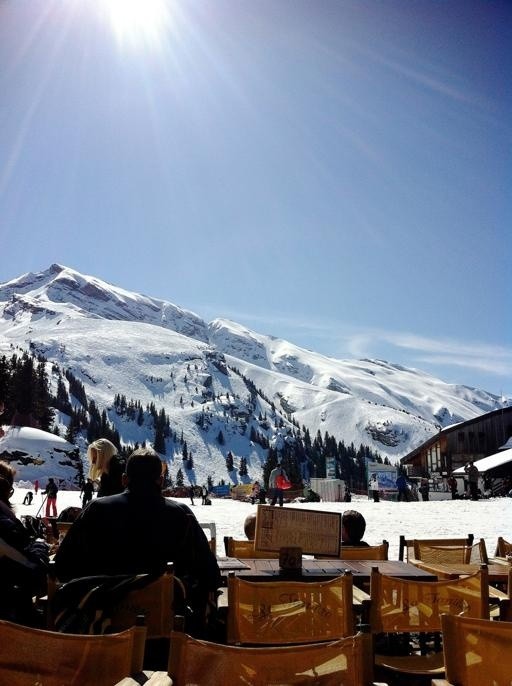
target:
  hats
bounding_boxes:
[125,448,168,480]
[341,509,366,533]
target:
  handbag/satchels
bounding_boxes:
[275,474,292,491]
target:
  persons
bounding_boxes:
[198,485,208,505]
[22,492,33,505]
[249,463,380,507]
[341,509,371,547]
[244,514,256,540]
[0,461,51,629]
[394,460,511,502]
[189,484,195,505]
[34,479,39,495]
[79,478,94,509]
[56,438,127,522]
[51,448,222,670]
[40,477,59,516]
